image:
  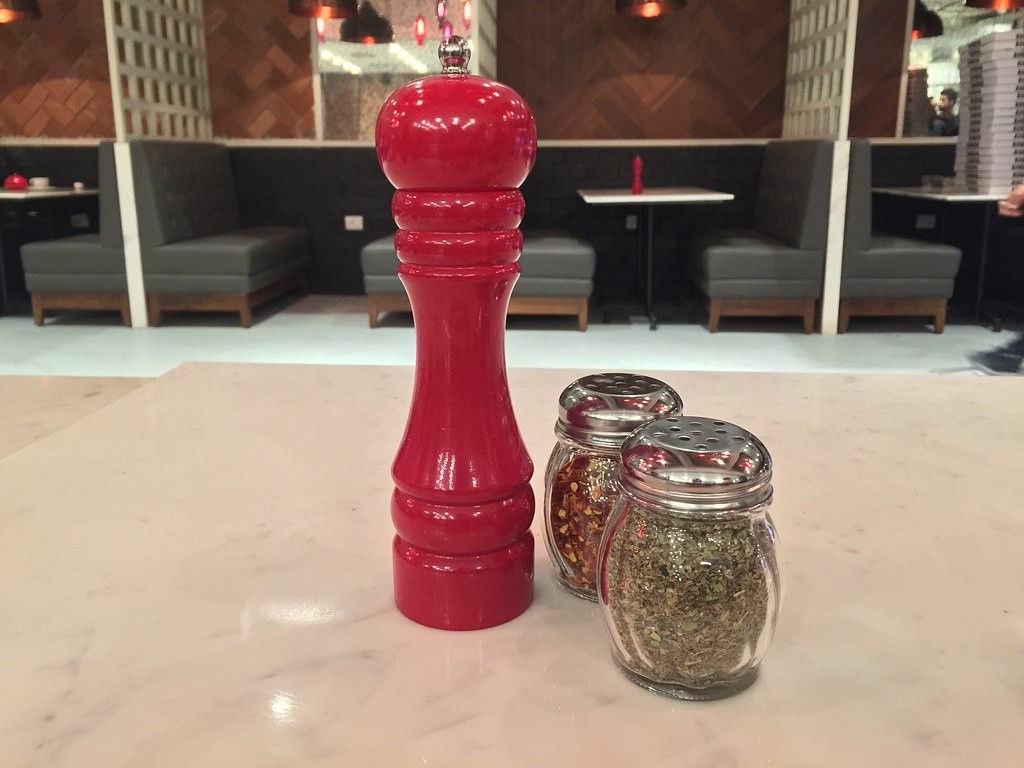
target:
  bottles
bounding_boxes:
[596,416,784,702]
[540,372,683,604]
[631,154,644,194]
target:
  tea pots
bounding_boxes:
[5,173,27,191]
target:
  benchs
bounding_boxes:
[682,137,962,335]
[361,231,596,331]
[20,139,316,329]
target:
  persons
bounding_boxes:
[970,180,1024,380]
[931,87,959,179]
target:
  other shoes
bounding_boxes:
[968,337,1024,375]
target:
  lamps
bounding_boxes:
[615,0,688,22]
[288,0,357,18]
[0,0,42,24]
[339,0,393,44]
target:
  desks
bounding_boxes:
[0,360,1024,768]
[0,186,100,315]
[576,187,735,331]
[871,185,1012,326]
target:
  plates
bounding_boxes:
[29,186,55,189]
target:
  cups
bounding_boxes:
[30,177,49,186]
